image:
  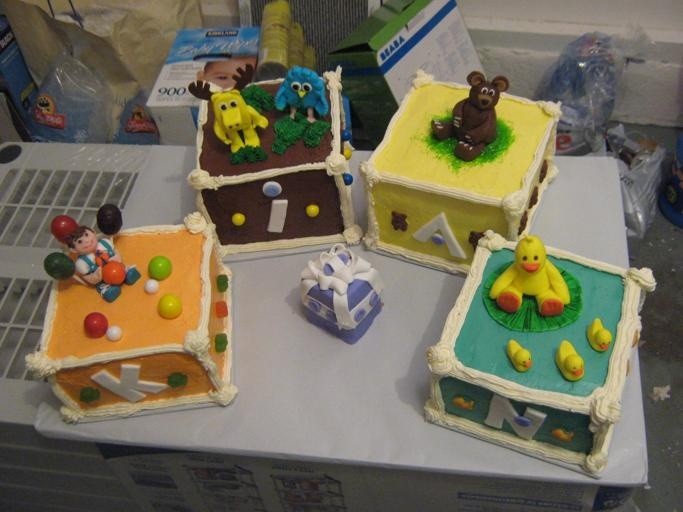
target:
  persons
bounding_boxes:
[204,58,257,89]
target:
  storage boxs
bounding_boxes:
[142,25,278,143]
[327,0,495,149]
